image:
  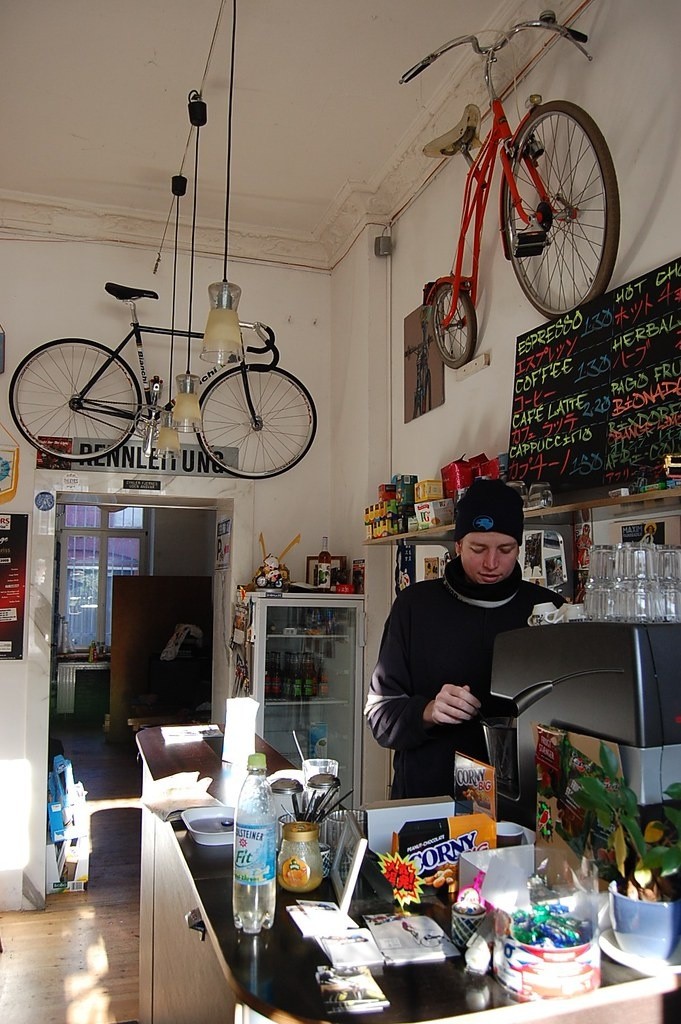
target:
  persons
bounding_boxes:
[364,480,559,800]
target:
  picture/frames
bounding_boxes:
[329,812,387,915]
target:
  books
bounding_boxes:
[286,902,461,1015]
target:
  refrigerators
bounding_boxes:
[235,590,365,810]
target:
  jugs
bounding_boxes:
[321,812,365,848]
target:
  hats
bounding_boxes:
[453,479,524,546]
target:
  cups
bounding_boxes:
[451,902,487,947]
[507,481,552,509]
[318,842,355,886]
[302,759,338,788]
[495,822,523,848]
[482,715,517,784]
[527,541,681,627]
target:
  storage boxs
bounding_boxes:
[359,751,601,1002]
[364,452,507,539]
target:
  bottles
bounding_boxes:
[316,536,331,593]
[276,820,322,892]
[307,774,340,814]
[271,778,304,851]
[88,641,108,662]
[233,753,277,933]
[264,648,329,701]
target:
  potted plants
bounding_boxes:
[556,731,681,961]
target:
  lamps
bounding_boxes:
[172,88,203,434]
[199,0,245,367]
[155,171,182,459]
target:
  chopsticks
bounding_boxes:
[280,783,354,825]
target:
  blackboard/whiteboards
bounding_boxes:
[507,257,681,493]
[621,521,665,545]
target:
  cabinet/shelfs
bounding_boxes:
[56,662,111,720]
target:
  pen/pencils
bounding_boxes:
[291,784,354,823]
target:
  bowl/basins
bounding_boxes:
[180,807,236,845]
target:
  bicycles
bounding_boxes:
[398,10,623,371]
[7,281,318,479]
